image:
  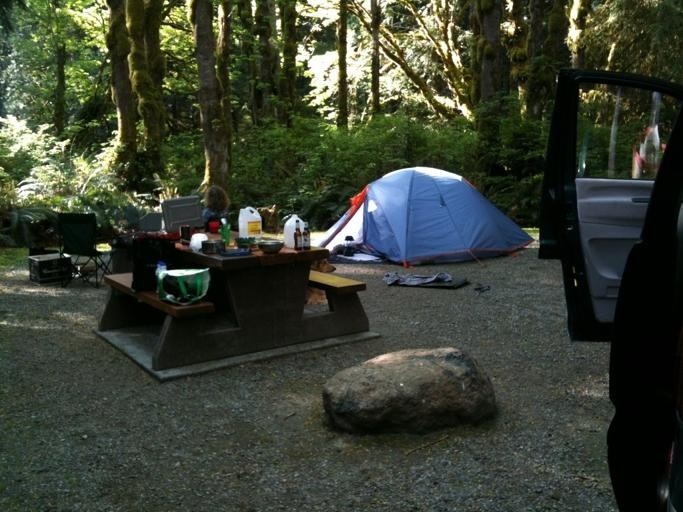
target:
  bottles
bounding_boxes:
[294,218,311,251]
[154,262,167,301]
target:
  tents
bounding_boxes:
[314,166,536,269]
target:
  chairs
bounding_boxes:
[56,211,114,288]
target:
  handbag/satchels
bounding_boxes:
[132,232,166,292]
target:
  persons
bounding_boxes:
[200,184,230,227]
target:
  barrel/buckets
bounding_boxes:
[238,207,262,239]
[284,214,305,248]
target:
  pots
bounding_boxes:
[201,239,225,255]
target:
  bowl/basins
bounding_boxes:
[257,241,285,254]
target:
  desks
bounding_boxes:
[171,226,330,354]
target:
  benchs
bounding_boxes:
[309,267,371,340]
[98,269,214,371]
